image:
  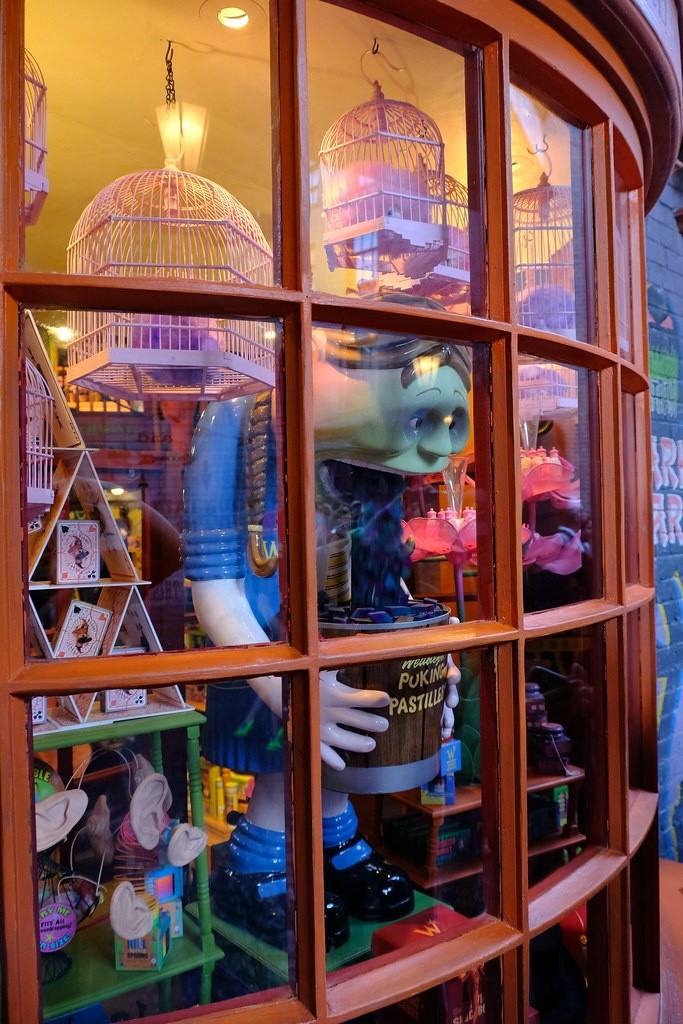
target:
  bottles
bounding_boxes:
[523,681,548,727]
[539,722,571,774]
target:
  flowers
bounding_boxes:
[401,446,581,789]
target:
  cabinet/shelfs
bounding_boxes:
[42,411,195,905]
[32,710,225,1021]
[372,763,586,891]
